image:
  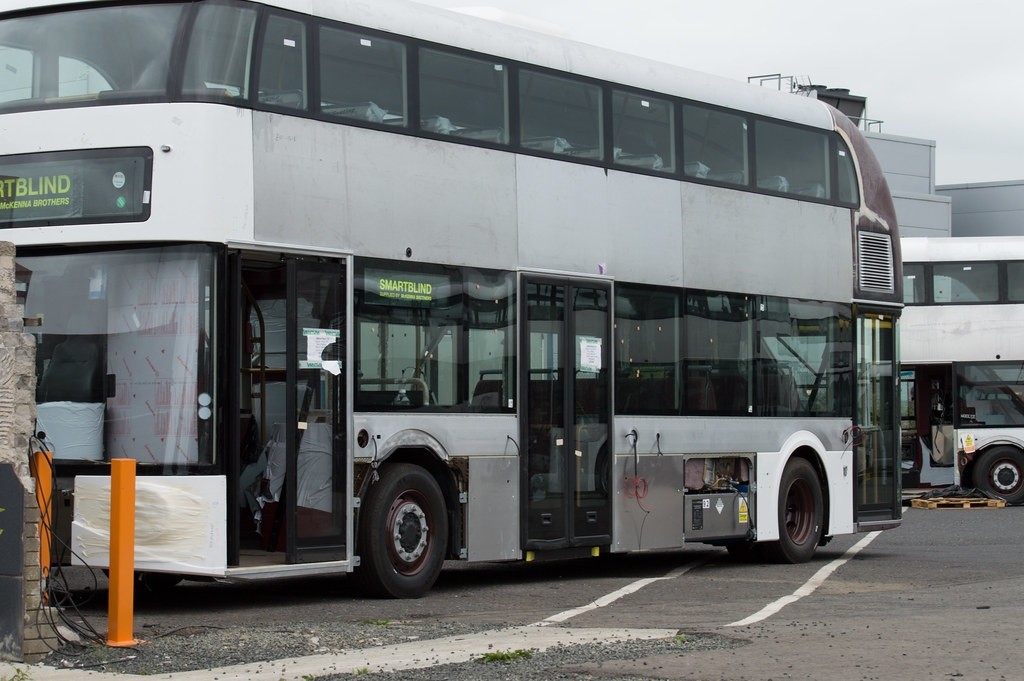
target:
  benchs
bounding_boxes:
[362,365,798,408]
[257,91,825,199]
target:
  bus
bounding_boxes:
[1,4,906,597]
[761,236,1024,506]
